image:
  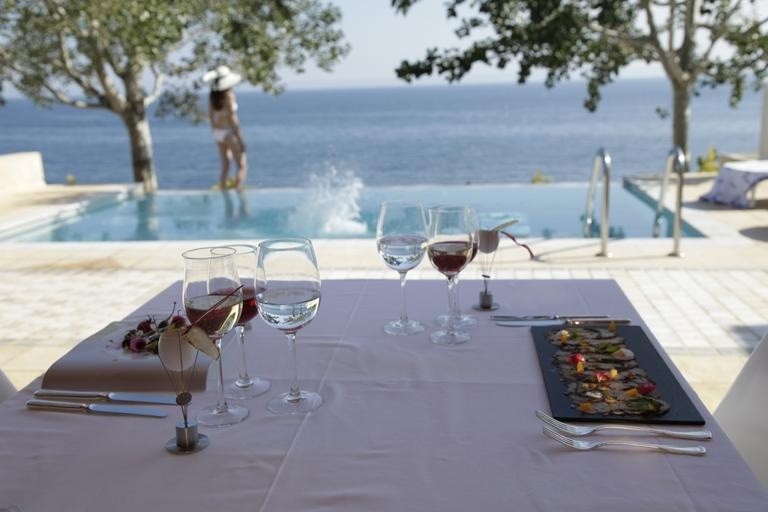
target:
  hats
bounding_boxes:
[202,65,244,92]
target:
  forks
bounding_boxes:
[535,409,714,455]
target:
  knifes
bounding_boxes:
[489,314,633,327]
[26,389,181,418]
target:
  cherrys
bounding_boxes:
[122,300,185,353]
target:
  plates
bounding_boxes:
[39,320,234,394]
[528,321,706,424]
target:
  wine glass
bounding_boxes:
[375,201,474,347]
[181,237,322,428]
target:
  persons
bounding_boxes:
[209,65,248,189]
